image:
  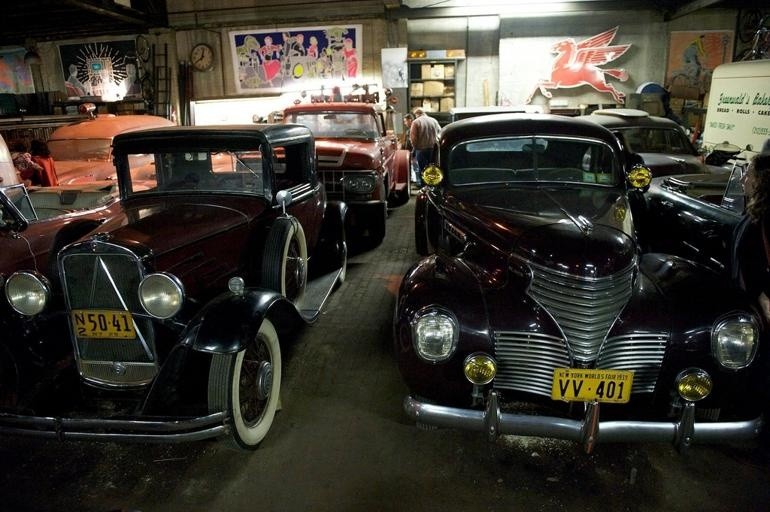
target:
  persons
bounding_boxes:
[20,139,59,187]
[11,140,43,185]
[730,155,770,333]
[410,108,442,191]
[400,114,422,190]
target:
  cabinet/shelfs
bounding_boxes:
[408,58,458,124]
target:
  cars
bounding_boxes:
[645,166,752,287]
[0,182,156,285]
[21,102,191,191]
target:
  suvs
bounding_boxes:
[389,111,769,445]
[0,127,348,453]
[576,109,734,199]
[234,101,409,239]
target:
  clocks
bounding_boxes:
[189,43,216,73]
[135,35,151,64]
